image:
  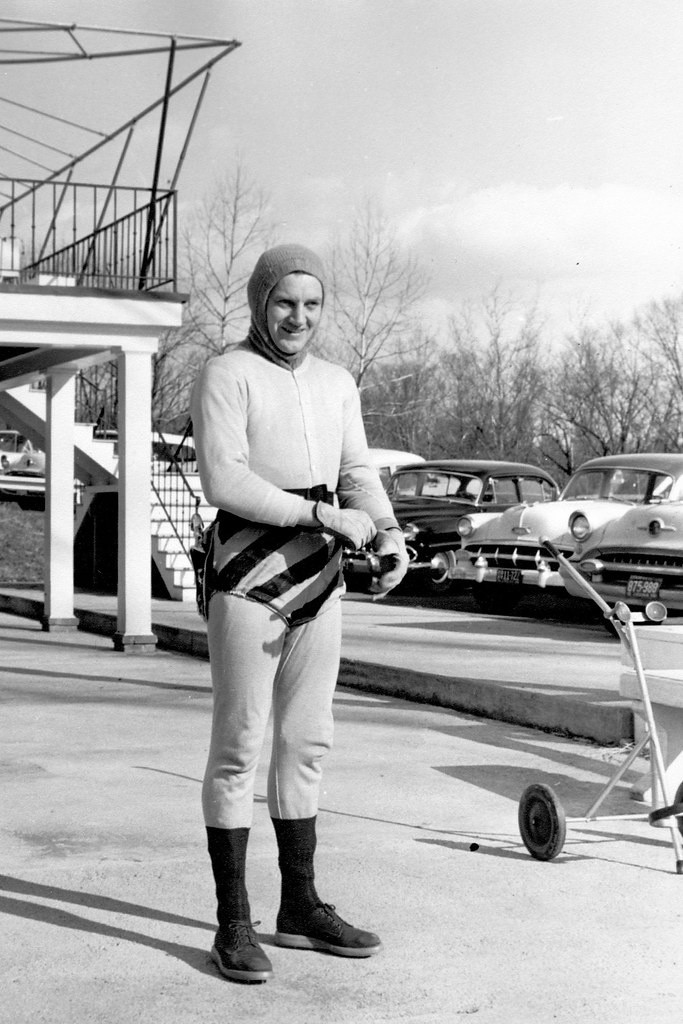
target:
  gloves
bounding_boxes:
[315,495,378,552]
[368,525,410,601]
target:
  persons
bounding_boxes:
[192,243,413,983]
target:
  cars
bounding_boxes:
[448,453,683,610]
[559,494,683,617]
[368,448,425,485]
[341,458,559,596]
[152,431,199,474]
[0,429,47,476]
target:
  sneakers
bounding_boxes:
[209,917,274,980]
[273,900,382,957]
